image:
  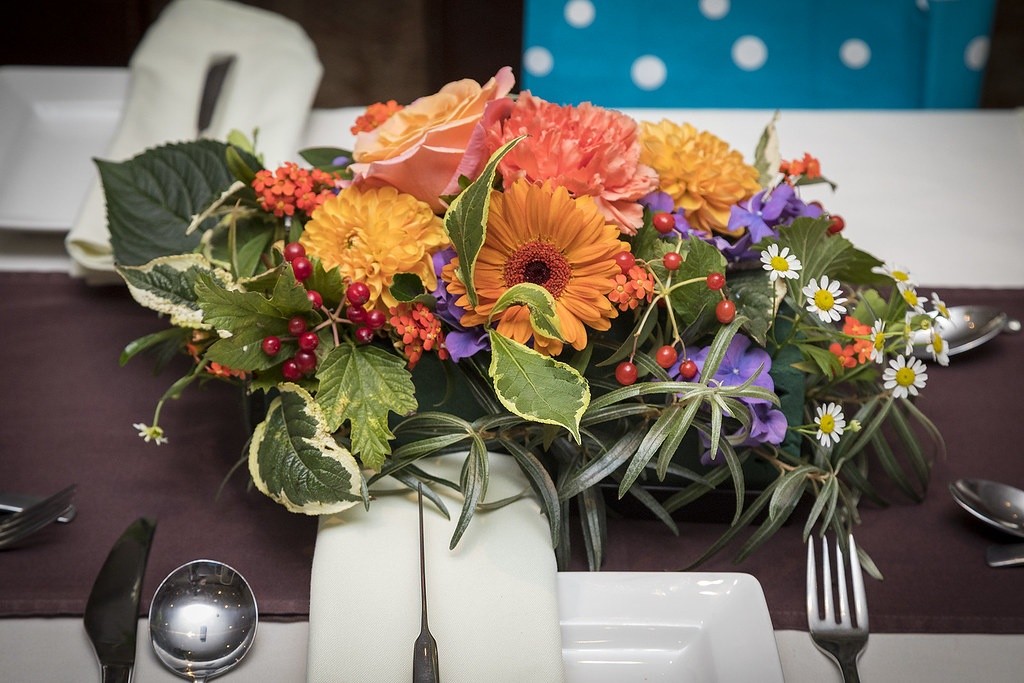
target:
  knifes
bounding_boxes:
[80,515,157,683]
[1,489,75,522]
[987,544,1024,567]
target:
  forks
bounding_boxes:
[805,534,870,683]
[0,482,76,547]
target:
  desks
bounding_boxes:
[0,109,1024,683]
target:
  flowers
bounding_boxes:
[85,64,978,572]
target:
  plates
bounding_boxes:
[553,570,783,683]
[0,65,125,234]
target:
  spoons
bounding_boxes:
[950,477,1024,535]
[909,304,1008,359]
[147,560,258,683]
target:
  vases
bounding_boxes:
[305,452,568,683]
